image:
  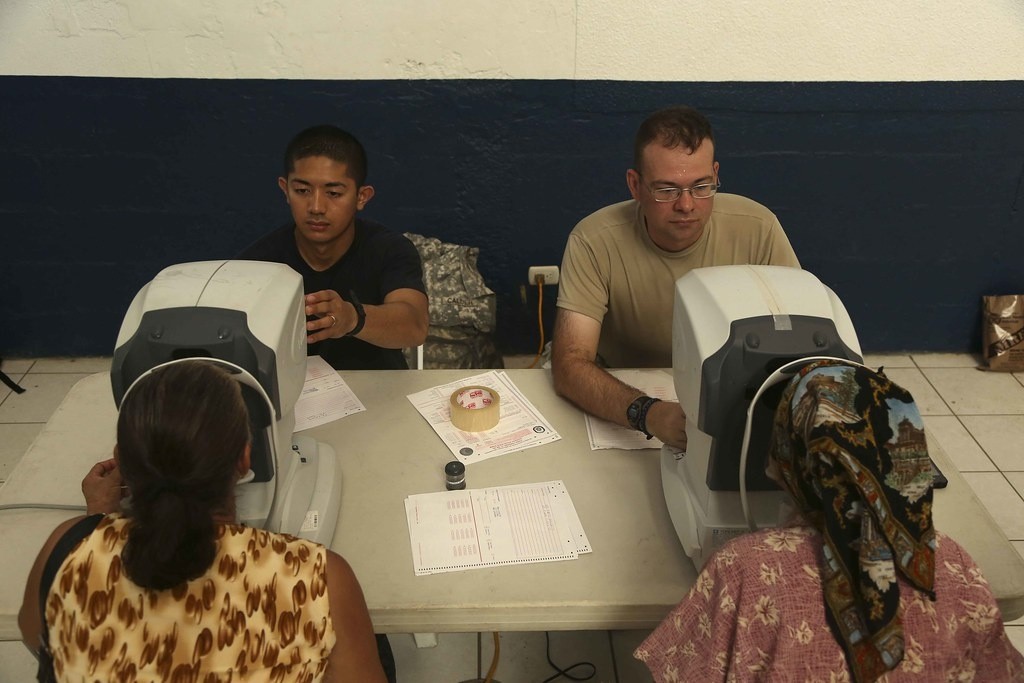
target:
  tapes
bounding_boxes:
[450,385,501,431]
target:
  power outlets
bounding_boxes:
[528,265,559,286]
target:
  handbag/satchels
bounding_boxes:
[976,294,1024,372]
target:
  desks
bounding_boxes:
[0,367,1024,649]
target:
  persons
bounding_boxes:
[17,359,386,682]
[551,104,801,453]
[231,124,430,372]
[632,357,1024,682]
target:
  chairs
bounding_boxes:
[402,232,505,369]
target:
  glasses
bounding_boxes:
[639,174,721,202]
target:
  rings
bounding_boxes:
[329,314,337,326]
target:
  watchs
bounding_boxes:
[627,395,654,441]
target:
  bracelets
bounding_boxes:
[640,398,661,436]
[346,289,366,338]
